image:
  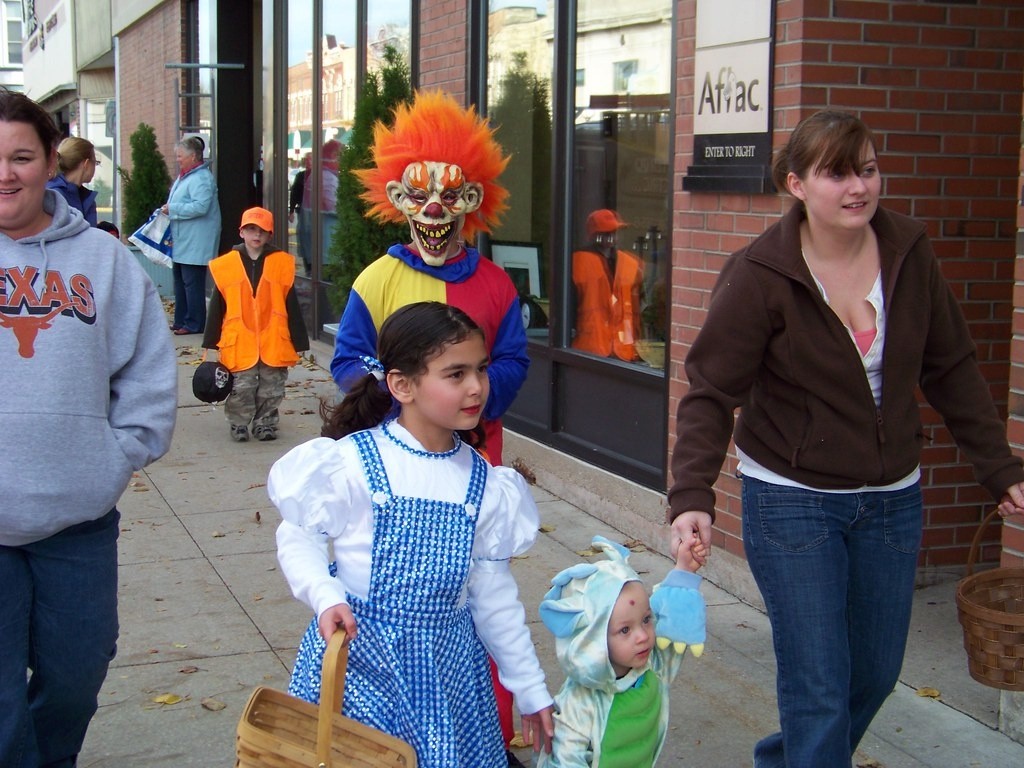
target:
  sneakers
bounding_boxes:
[231,425,249,441]
[253,425,277,440]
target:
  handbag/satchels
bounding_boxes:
[127,208,174,269]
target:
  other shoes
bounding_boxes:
[306,270,312,279]
[170,325,180,329]
[174,328,189,334]
[505,749,525,768]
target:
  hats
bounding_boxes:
[322,141,341,171]
[239,207,273,234]
[586,210,628,236]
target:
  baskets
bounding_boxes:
[955,504,1024,691]
[236,629,416,768]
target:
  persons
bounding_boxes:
[531,534,707,768]
[202,207,310,442]
[46,137,100,229]
[331,86,531,767]
[0,86,179,768]
[566,208,648,367]
[290,141,345,279]
[267,301,555,768]
[161,136,222,336]
[668,107,1024,767]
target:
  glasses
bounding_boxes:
[85,157,100,166]
